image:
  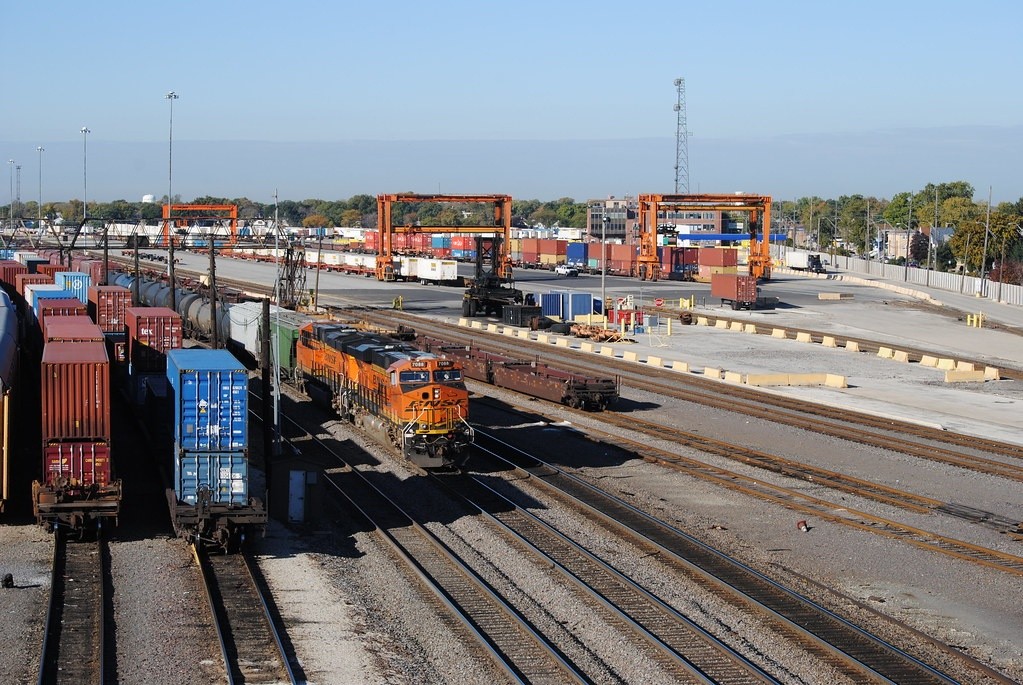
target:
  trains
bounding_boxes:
[185,236,458,289]
[293,229,741,285]
[0,243,475,553]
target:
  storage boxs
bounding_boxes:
[217,231,757,303]
[0,246,251,507]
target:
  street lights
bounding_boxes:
[163,91,180,277]
[822,216,838,267]
[79,126,91,247]
[35,146,45,234]
[896,222,911,282]
[7,159,15,229]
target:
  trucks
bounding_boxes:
[785,251,826,274]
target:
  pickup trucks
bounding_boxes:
[555,264,578,277]
